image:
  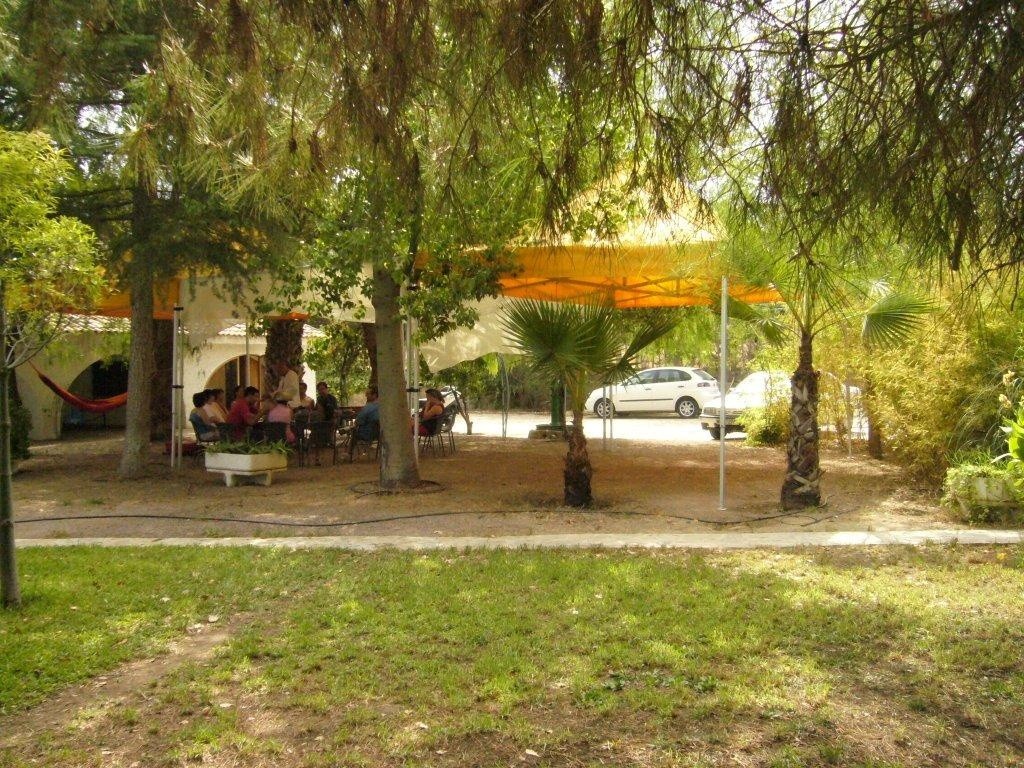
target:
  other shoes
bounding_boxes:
[314,461,322,466]
[339,427,348,435]
[338,454,349,463]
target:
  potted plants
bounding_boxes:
[201,437,295,488]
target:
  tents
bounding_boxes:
[412,161,853,509]
[1,211,411,468]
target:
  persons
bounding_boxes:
[191,360,447,462]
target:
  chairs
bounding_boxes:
[188,405,459,469]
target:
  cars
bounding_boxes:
[699,367,848,441]
[584,367,721,419]
